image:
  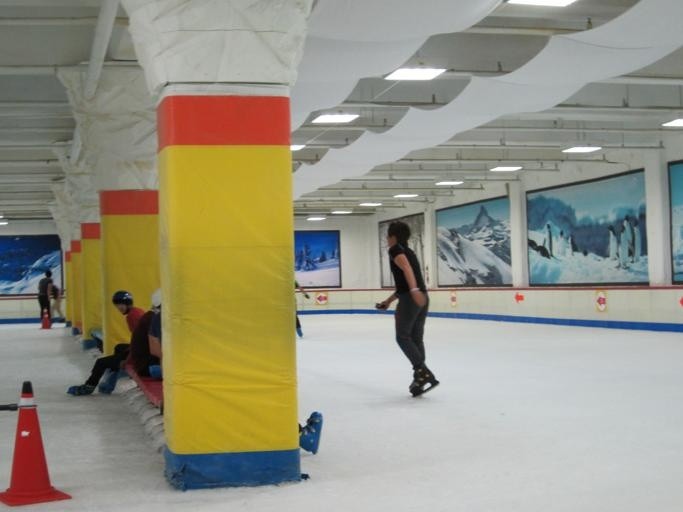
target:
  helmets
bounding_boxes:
[112,290,133,303]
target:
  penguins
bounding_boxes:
[606,212,646,271]
[527,223,588,259]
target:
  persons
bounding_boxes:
[296,282,309,328]
[130,290,160,379]
[376,222,433,390]
[147,312,160,377]
[70,291,144,396]
[51,285,65,321]
[39,271,53,321]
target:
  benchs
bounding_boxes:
[76,321,163,408]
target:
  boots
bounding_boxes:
[409,363,434,391]
[99,371,120,391]
[68,380,96,394]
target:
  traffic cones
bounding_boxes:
[0,380,71,507]
[41,308,52,330]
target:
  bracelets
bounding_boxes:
[409,288,419,292]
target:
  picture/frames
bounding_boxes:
[666,159,683,285]
[378,211,425,290]
[293,230,342,288]
[524,165,650,287]
[0,234,64,296]
[435,194,513,288]
